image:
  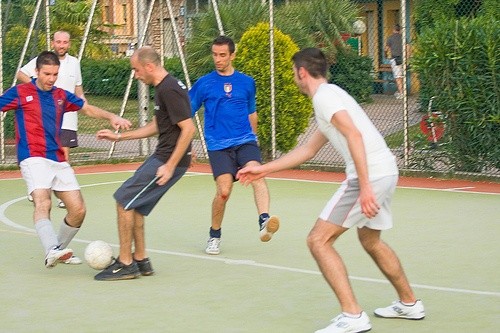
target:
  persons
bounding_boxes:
[384,24,410,100]
[0,30,132,269]
[94,45,196,281]
[188,35,280,255]
[236,47,426,333]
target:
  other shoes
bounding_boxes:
[57,199,66,208]
[28,195,33,202]
[394,91,404,99]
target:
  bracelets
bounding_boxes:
[118,133,122,141]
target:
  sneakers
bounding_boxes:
[95,255,137,280]
[63,255,81,264]
[373,298,425,319]
[259,215,279,242]
[131,253,154,275]
[205,236,221,254]
[314,310,372,333]
[44,243,73,269]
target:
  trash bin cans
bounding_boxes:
[418,95,456,150]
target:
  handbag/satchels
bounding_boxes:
[395,55,402,64]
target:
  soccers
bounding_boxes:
[84,240,114,270]
